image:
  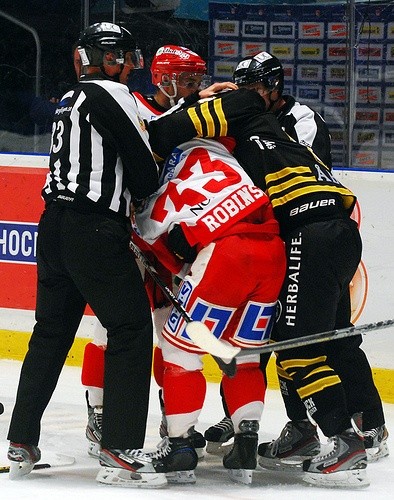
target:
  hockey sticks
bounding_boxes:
[128,240,236,378]
[184,319,394,358]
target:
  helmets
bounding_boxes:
[77,22,141,68]
[231,51,285,90]
[151,44,206,91]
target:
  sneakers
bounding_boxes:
[7,442,41,479]
[144,425,198,484]
[158,389,206,459]
[257,409,321,472]
[222,419,259,486]
[299,413,368,488]
[204,415,235,452]
[86,390,103,460]
[95,446,167,488]
[362,423,389,461]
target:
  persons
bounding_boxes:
[4,24,165,485]
[83,45,220,459]
[112,118,287,482]
[146,85,389,490]
[216,47,347,465]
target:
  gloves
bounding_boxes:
[163,223,197,265]
